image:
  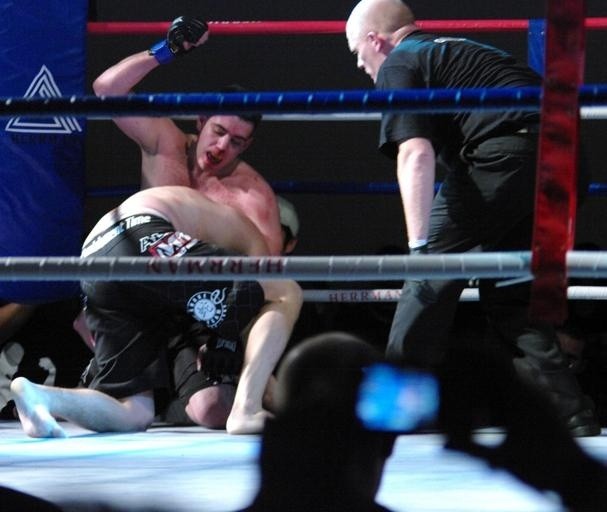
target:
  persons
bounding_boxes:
[341,0,593,433]
[292,245,409,352]
[235,330,393,512]
[568,245,607,427]
[90,9,285,428]
[3,306,85,425]
[11,186,303,437]
[433,364,604,512]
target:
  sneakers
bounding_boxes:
[558,406,604,439]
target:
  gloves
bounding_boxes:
[404,241,440,311]
[200,328,245,386]
[167,14,210,57]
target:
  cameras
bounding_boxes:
[356,359,439,434]
